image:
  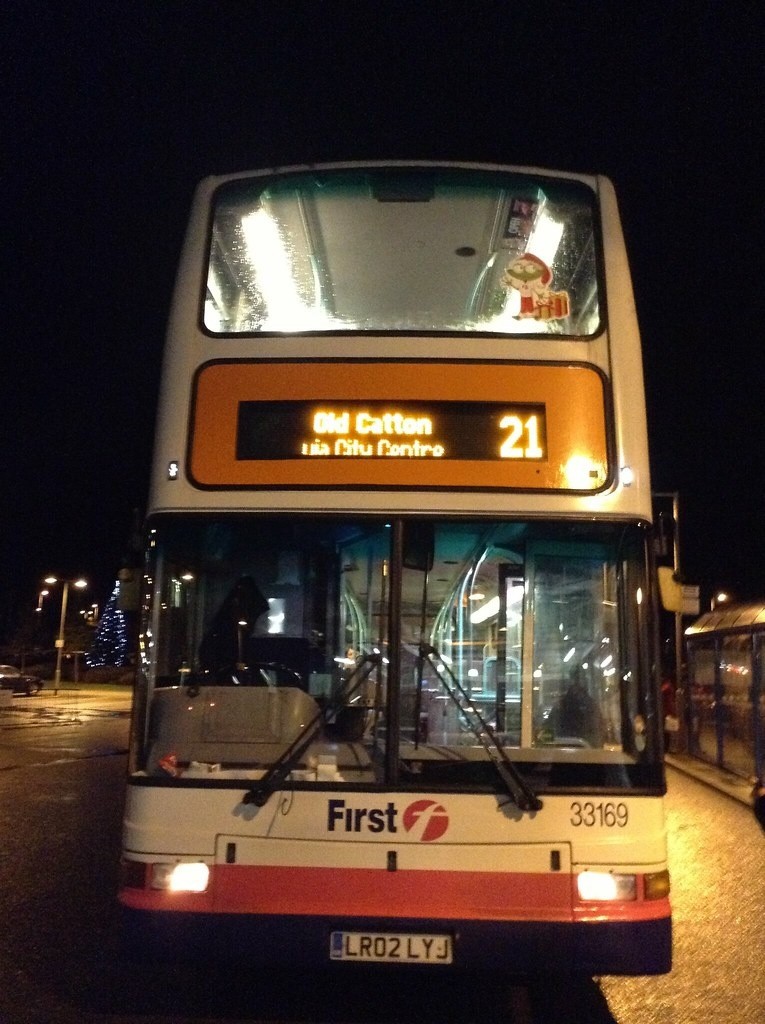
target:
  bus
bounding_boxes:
[114,156,688,978]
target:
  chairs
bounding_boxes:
[481,657,523,722]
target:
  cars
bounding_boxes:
[0,665,44,696]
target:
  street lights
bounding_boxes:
[710,592,727,610]
[238,620,248,665]
[44,575,89,696]
